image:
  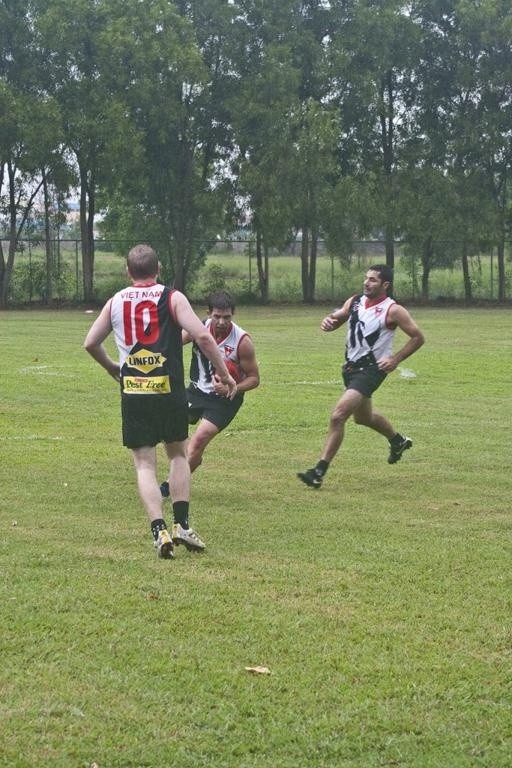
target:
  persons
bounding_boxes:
[296,264,425,489]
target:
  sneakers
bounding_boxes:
[153,523,205,558]
[388,437,413,464]
[160,482,169,497]
[296,469,322,488]
